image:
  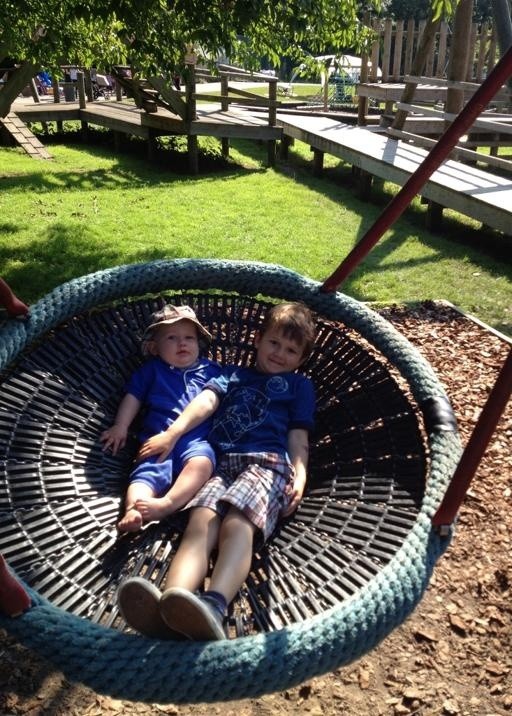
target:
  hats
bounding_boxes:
[140,304,212,356]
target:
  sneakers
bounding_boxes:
[117,577,189,642]
[159,587,226,641]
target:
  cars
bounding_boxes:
[289,54,382,86]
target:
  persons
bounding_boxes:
[116,302,316,642]
[98,304,222,535]
